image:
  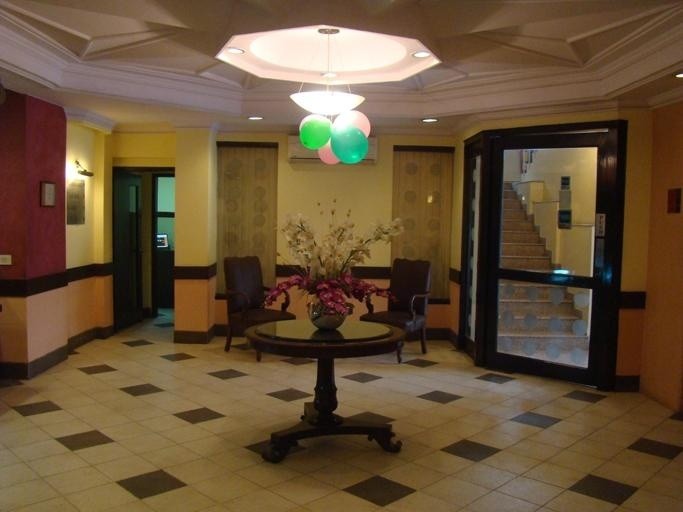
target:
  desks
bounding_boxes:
[245,318,406,463]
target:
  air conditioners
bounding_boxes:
[287,135,379,164]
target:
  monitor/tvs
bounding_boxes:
[156,233,168,248]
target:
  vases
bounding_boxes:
[307,294,345,329]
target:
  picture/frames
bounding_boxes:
[40,180,57,207]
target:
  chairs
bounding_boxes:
[360,257,432,364]
[224,256,296,360]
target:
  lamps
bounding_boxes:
[75,160,94,177]
[289,29,365,116]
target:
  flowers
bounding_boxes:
[264,197,406,312]
